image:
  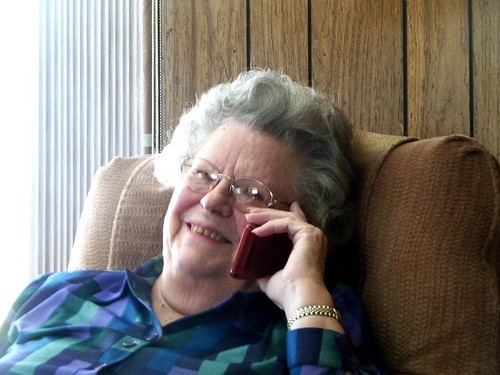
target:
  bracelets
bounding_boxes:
[281,304,343,329]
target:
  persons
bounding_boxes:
[0,66,386,375]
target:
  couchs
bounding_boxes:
[67,130,500,375]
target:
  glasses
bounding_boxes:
[179,160,303,212]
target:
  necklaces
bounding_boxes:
[156,281,192,318]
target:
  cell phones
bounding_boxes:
[230,224,293,278]
